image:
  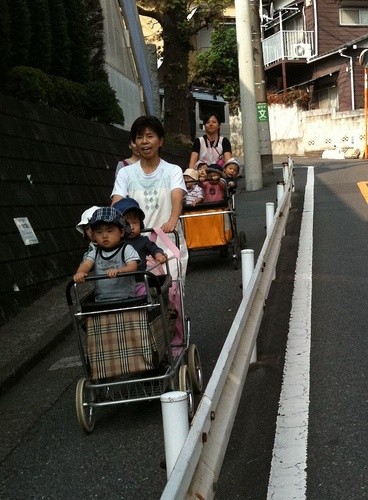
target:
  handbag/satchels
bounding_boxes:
[134,281,185,355]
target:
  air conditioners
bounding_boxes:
[291,44,311,58]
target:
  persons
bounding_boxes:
[73,198,167,302]
[184,158,240,206]
[115,138,140,176]
[111,115,189,360]
[189,114,232,256]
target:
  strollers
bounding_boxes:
[180,178,246,270]
[65,227,203,435]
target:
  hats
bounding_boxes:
[112,198,145,220]
[89,207,130,240]
[223,157,241,179]
[183,168,198,180]
[76,206,103,236]
